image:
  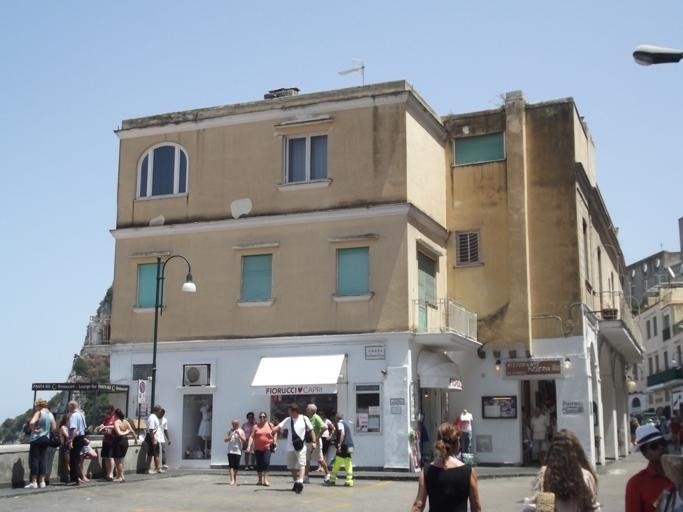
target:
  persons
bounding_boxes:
[411,423,481,512]
[224,402,354,494]
[198,405,211,441]
[459,409,473,451]
[22,397,172,489]
[530,406,548,465]
[626,400,683,512]
[522,428,602,512]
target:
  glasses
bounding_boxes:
[644,438,667,450]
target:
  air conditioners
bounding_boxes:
[183,364,209,386]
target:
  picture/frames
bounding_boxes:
[481,394,517,419]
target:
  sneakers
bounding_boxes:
[23,482,37,489]
[38,481,45,488]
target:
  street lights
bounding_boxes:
[149,253,197,412]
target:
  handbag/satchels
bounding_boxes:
[270,443,276,452]
[535,464,556,512]
[291,429,303,451]
[49,432,60,447]
[303,416,311,442]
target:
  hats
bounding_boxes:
[633,423,666,451]
